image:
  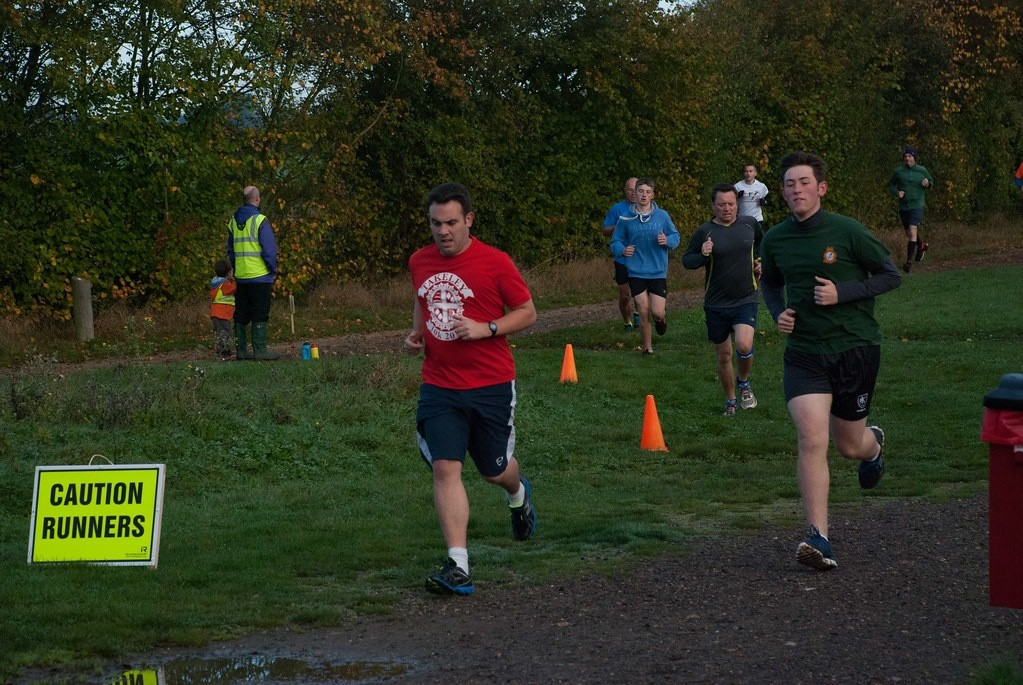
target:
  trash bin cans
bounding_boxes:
[982,372,1023,608]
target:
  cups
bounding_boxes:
[311,344,320,359]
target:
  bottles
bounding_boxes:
[301,341,311,360]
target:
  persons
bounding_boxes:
[404,182,537,597]
[682,184,764,417]
[601,179,640,329]
[758,151,903,575]
[1014,162,1023,191]
[208,256,237,357]
[226,186,279,361]
[895,146,936,274]
[732,163,773,267]
[610,177,681,358]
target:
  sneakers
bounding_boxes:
[632,312,639,328]
[724,399,737,415]
[652,313,666,335]
[425,557,474,596]
[641,349,654,356]
[735,377,758,409]
[915,243,929,261]
[903,263,912,272]
[507,476,536,541]
[624,322,633,329]
[859,426,885,488]
[796,524,837,571]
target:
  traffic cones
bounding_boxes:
[640,394,669,453]
[559,344,578,384]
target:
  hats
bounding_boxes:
[905,148,916,155]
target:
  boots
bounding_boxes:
[251,322,278,361]
[235,322,254,360]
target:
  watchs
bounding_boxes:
[488,321,497,338]
